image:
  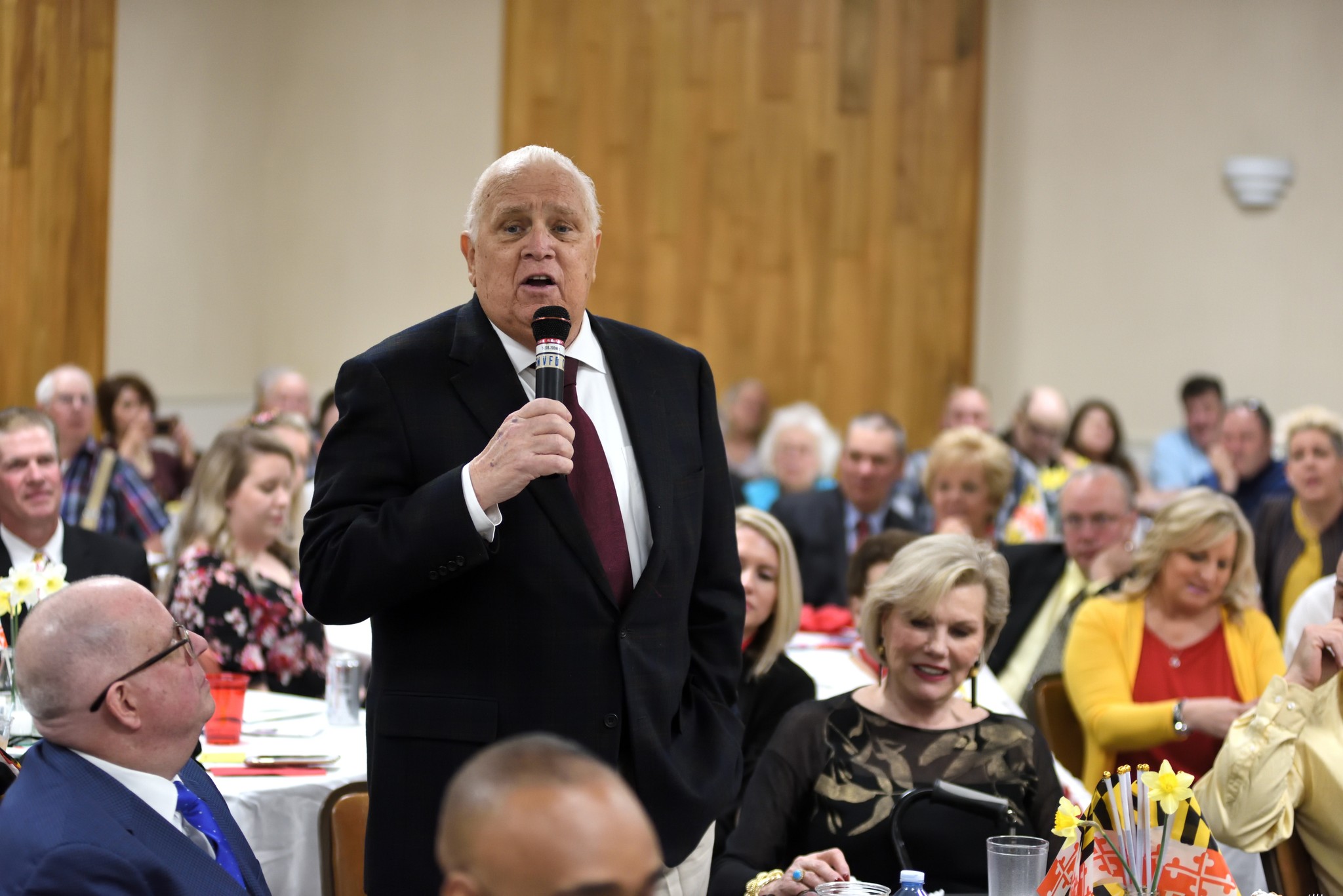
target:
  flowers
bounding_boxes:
[0,555,71,709]
[1049,756,1194,896]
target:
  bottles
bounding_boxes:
[892,870,928,896]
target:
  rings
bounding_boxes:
[793,870,805,881]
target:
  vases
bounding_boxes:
[0,646,16,747]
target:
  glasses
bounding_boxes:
[89,621,195,712]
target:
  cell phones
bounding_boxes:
[245,753,341,766]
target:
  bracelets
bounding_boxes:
[743,869,784,896]
[1172,698,1188,732]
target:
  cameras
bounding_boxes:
[154,416,173,432]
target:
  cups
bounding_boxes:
[986,835,1050,896]
[205,672,250,745]
[814,881,891,896]
[0,714,15,753]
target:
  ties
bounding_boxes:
[529,357,633,603]
[33,552,48,571]
[1019,589,1087,724]
[173,779,245,888]
[855,520,871,550]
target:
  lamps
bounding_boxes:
[1221,154,1289,210]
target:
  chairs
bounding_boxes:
[1032,672,1083,776]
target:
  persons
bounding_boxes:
[0,574,273,896]
[298,143,747,896]
[34,364,199,544]
[708,371,1343,896]
[433,730,666,896]
[155,426,330,697]
[250,369,342,481]
[0,407,151,645]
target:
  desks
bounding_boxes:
[2,687,368,896]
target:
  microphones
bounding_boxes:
[531,306,572,402]
